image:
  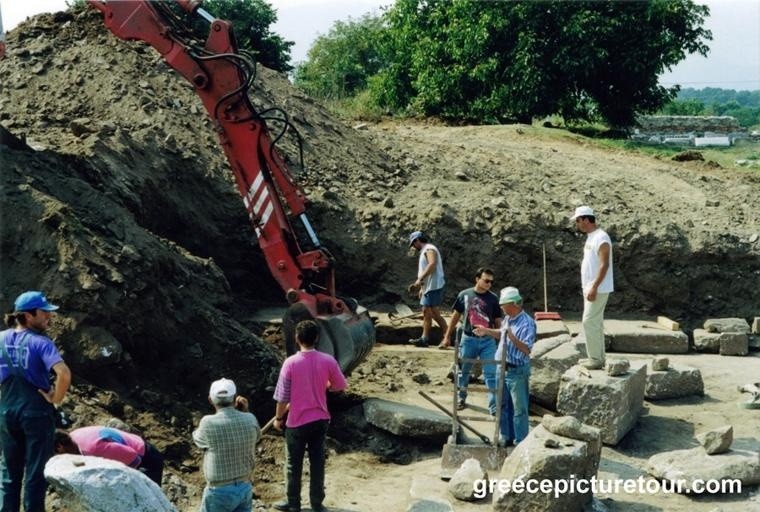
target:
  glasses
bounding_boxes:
[485,279,494,284]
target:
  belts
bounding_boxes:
[207,475,248,486]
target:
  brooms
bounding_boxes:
[532,244,562,321]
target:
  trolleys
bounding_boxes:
[438,328,509,485]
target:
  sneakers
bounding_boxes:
[580,359,605,369]
[273,501,300,512]
[409,338,427,346]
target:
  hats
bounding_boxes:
[210,378,237,397]
[14,291,60,311]
[569,206,594,221]
[408,231,426,248]
[500,287,522,307]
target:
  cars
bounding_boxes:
[632,131,760,147]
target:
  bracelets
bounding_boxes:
[419,276,423,281]
[276,416,283,421]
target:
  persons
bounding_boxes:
[409,231,452,347]
[439,268,503,384]
[54,426,164,487]
[273,320,348,512]
[192,378,262,512]
[472,287,536,447]
[0,291,71,512]
[570,206,614,369]
